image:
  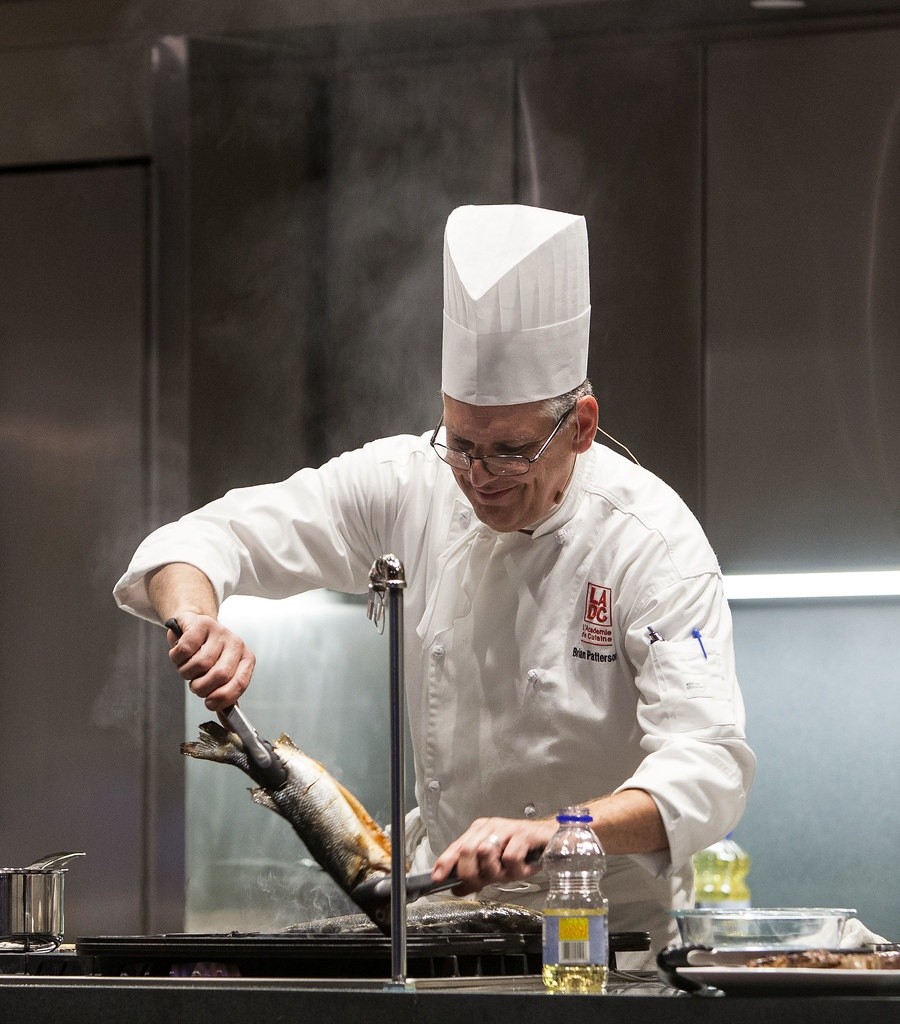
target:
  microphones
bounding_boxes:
[556,397,581,505]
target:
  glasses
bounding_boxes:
[430,392,599,476]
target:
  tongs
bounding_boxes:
[166,617,288,791]
[349,849,549,906]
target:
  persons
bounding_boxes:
[111,202,758,972]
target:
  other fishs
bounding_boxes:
[258,897,545,939]
[178,711,402,942]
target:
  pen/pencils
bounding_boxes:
[646,624,665,645]
[693,629,708,659]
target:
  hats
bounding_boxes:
[441,204,591,406]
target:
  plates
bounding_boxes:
[675,967,900,993]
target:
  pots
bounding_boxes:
[1,849,88,943]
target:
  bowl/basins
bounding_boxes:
[675,906,858,952]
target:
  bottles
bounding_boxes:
[693,827,753,916]
[539,808,610,991]
